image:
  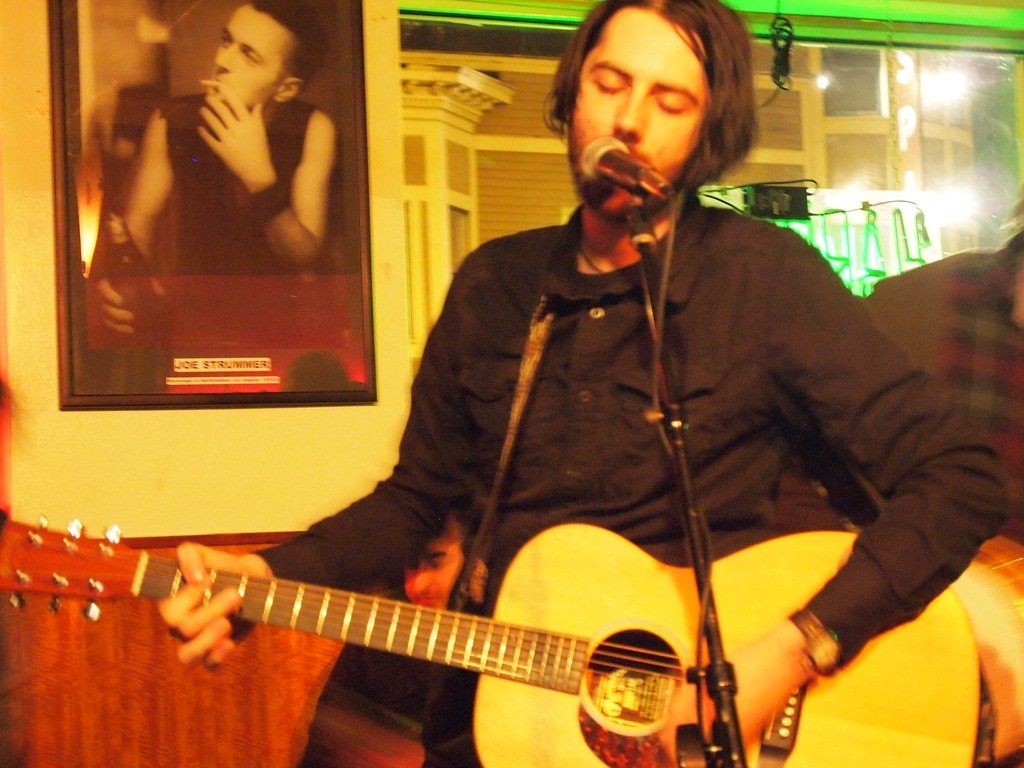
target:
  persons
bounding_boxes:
[96,1,336,336]
[856,183,1024,548]
[159,1,1007,768]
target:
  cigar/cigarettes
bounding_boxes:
[198,80,220,87]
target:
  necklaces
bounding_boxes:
[573,242,617,276]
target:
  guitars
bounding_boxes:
[0,519,982,768]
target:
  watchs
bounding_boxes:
[784,610,842,674]
